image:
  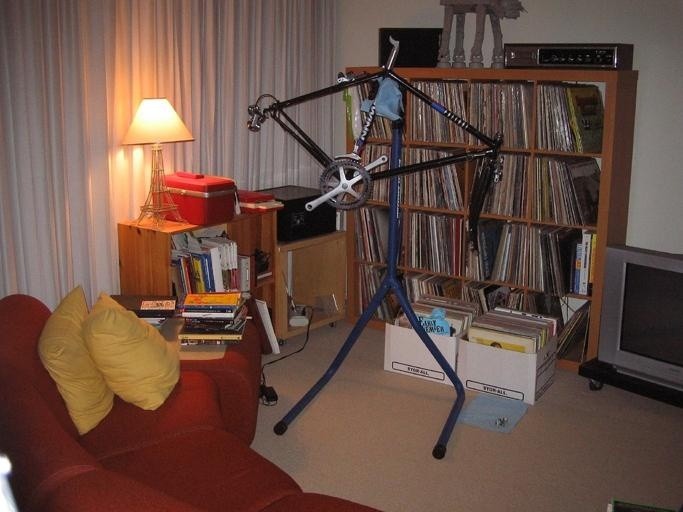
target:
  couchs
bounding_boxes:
[0,295,381,512]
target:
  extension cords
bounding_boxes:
[289,315,309,327]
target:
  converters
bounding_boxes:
[263,386,278,401]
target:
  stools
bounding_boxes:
[179,322,261,446]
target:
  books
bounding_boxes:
[356,143,601,225]
[606,501,681,512]
[359,261,592,362]
[137,217,254,349]
[355,204,595,296]
[250,295,281,355]
[354,80,603,152]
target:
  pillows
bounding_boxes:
[36,285,114,437]
[82,292,178,411]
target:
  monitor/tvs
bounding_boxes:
[597,241,683,394]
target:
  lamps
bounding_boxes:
[119,97,195,229]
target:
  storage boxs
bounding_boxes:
[255,185,336,241]
[152,172,235,225]
[384,320,466,387]
[456,333,560,406]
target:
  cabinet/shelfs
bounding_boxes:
[118,206,346,345]
[344,66,639,372]
